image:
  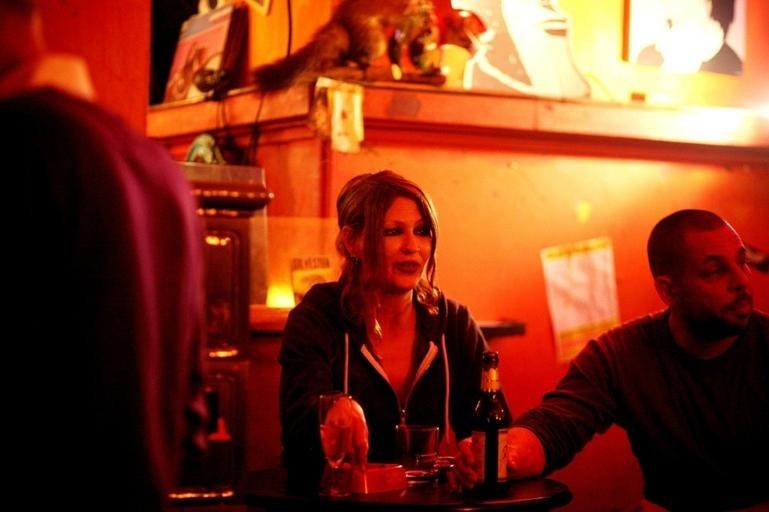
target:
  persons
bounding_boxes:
[279,171,511,491]
[447,208,769,510]
[0,0,209,509]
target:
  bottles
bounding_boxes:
[468,351,511,499]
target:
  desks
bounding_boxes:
[224,470,573,511]
[250,317,528,340]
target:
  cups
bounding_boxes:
[393,423,440,485]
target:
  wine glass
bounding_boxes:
[317,392,352,497]
